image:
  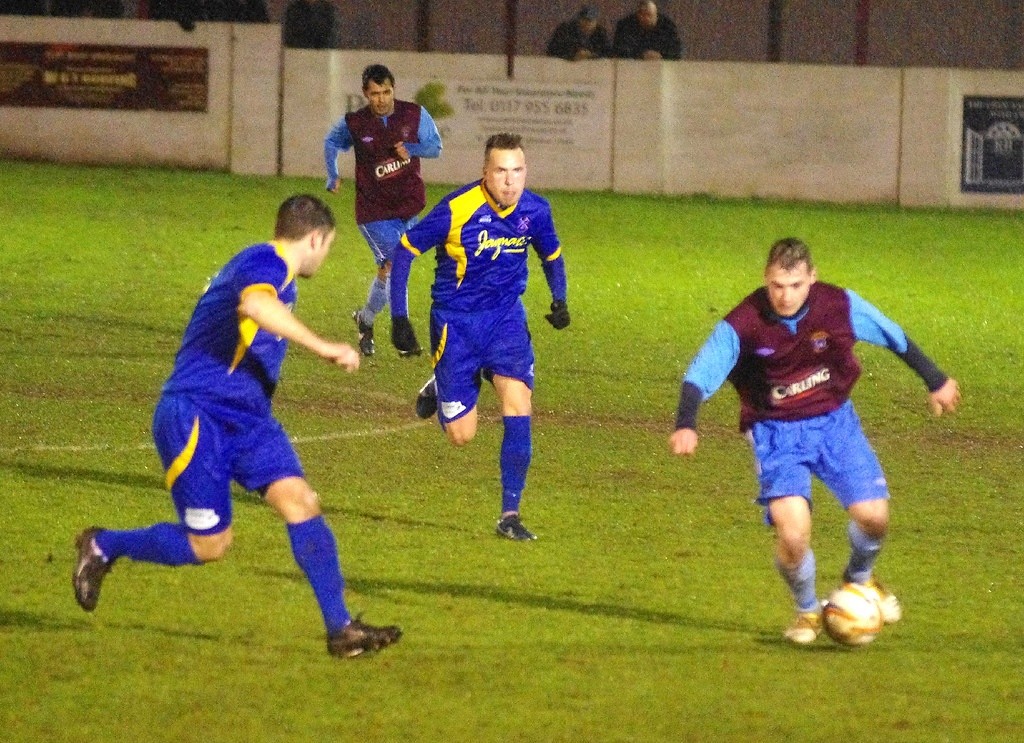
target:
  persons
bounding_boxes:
[389,133,570,540]
[324,64,443,356]
[547,5,612,64]
[73,194,403,660]
[615,0,683,61]
[669,239,960,647]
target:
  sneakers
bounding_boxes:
[415,374,438,419]
[352,310,375,357]
[497,513,538,541]
[72,526,116,613]
[786,602,823,643]
[399,347,420,356]
[327,612,404,659]
[843,577,903,624]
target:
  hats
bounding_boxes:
[579,6,597,19]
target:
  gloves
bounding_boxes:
[391,315,415,350]
[545,300,570,330]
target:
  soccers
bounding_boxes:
[822,582,885,646]
